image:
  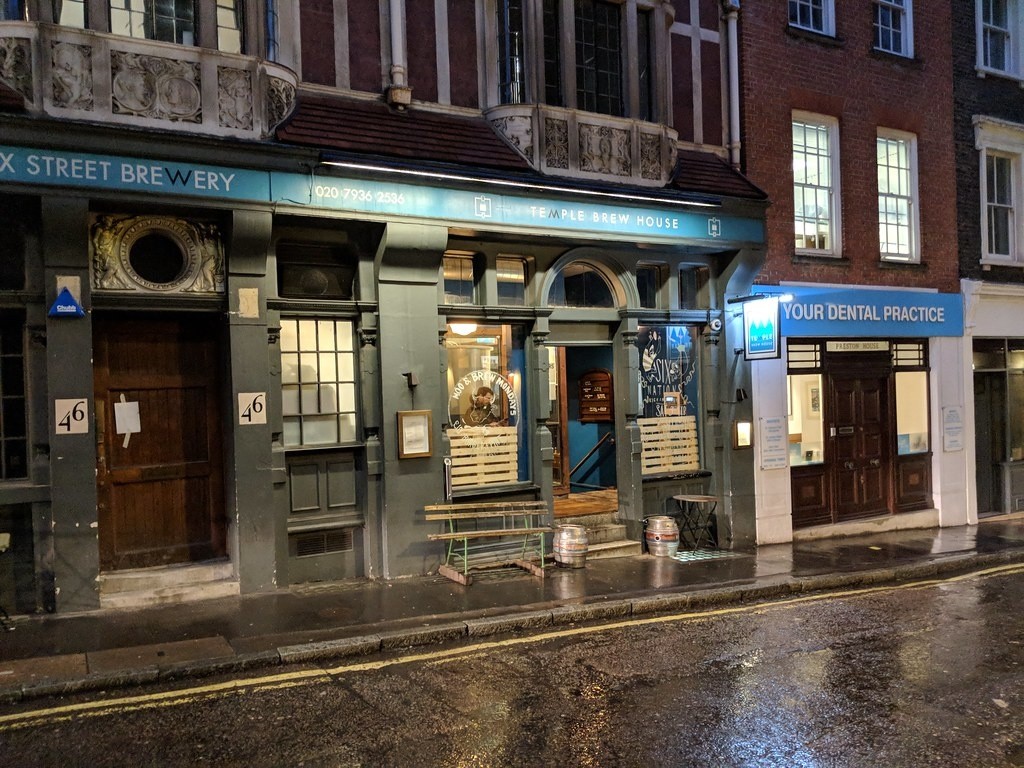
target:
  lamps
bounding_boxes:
[721,388,749,404]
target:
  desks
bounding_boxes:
[673,494,721,556]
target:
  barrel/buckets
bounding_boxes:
[552,524,588,568]
[646,516,680,557]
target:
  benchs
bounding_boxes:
[424,502,564,585]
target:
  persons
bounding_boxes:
[461,386,500,428]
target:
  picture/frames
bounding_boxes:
[397,410,434,458]
